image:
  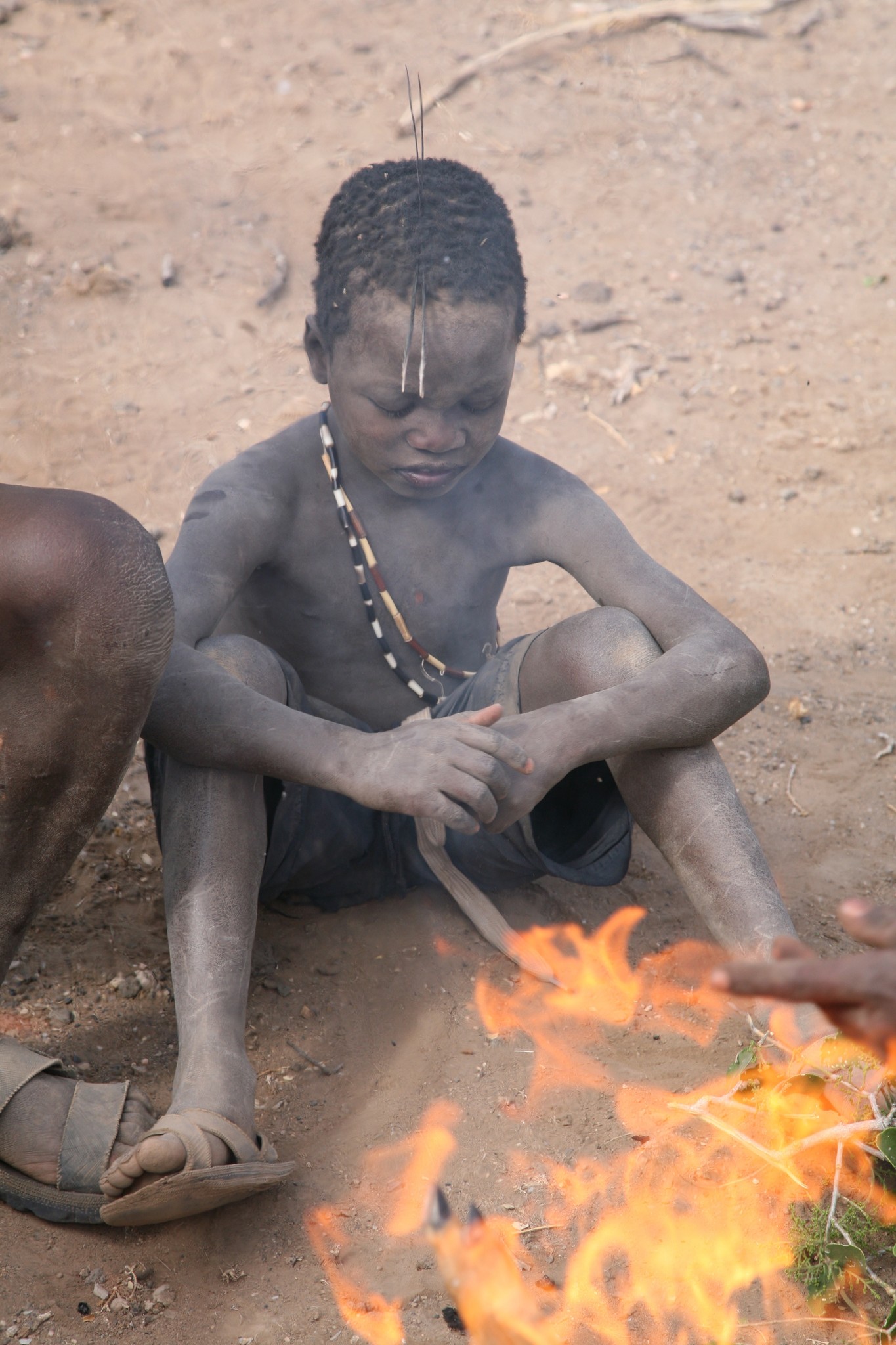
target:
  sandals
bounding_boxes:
[0,1031,167,1225]
[98,1109,296,1226]
[786,1028,895,1140]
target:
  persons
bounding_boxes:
[4,482,177,1227]
[106,157,824,1233]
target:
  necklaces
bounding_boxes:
[318,413,502,706]
